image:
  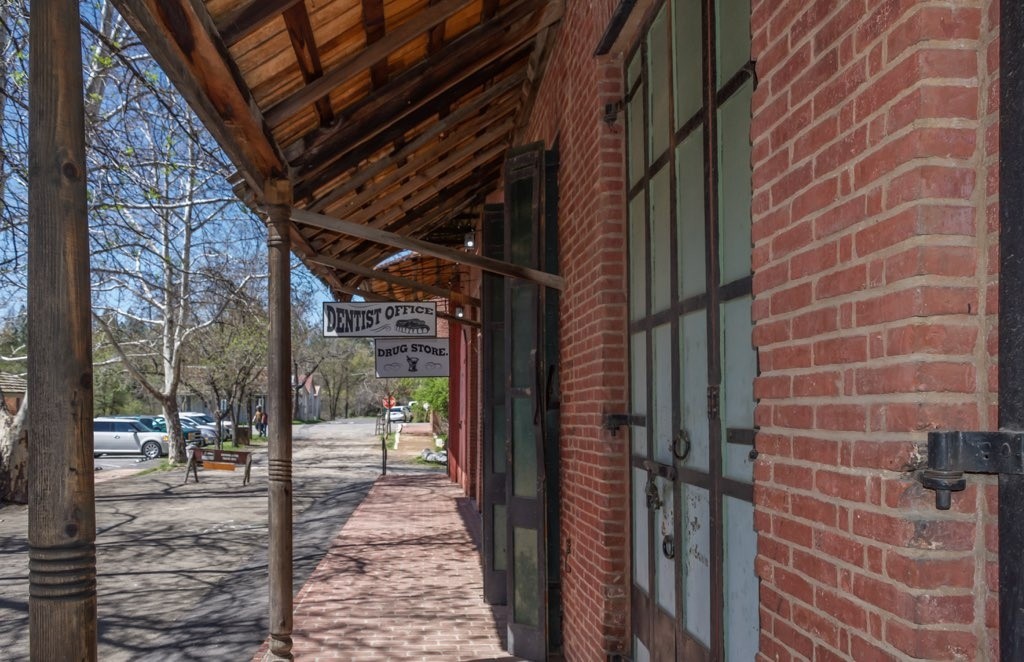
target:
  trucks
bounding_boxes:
[408,401,430,410]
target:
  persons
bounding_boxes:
[250,405,264,437]
[261,412,268,436]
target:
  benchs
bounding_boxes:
[184,448,252,486]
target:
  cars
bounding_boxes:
[92,412,232,459]
[390,406,412,423]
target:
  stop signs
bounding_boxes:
[383,395,396,408]
